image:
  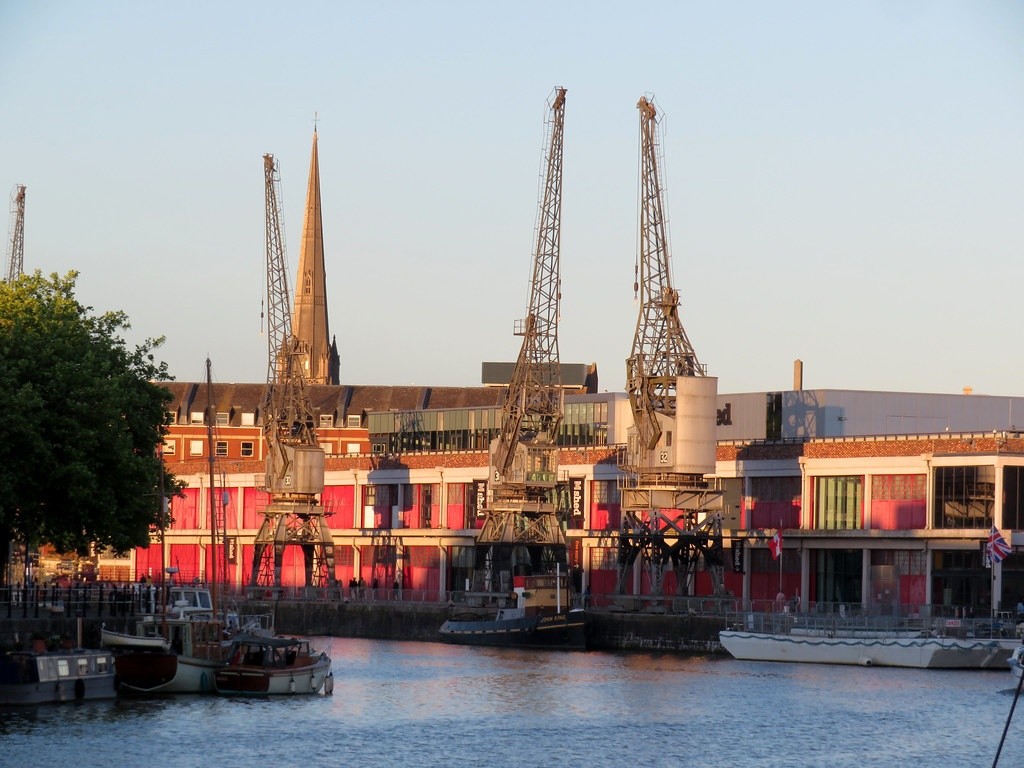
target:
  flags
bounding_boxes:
[767,529,782,560]
[986,524,1012,564]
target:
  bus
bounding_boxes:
[445,541,571,610]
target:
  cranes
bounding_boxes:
[466,85,576,605]
[246,153,343,588]
[586,99,724,599]
[9,185,26,283]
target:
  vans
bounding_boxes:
[167,587,213,613]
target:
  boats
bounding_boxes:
[439,563,597,650]
[1,358,336,706]
[717,624,1015,670]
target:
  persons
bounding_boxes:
[392,579,399,601]
[140,574,151,590]
[372,578,378,600]
[777,589,798,613]
[1016,598,1024,624]
[349,578,365,599]
[51,575,57,586]
